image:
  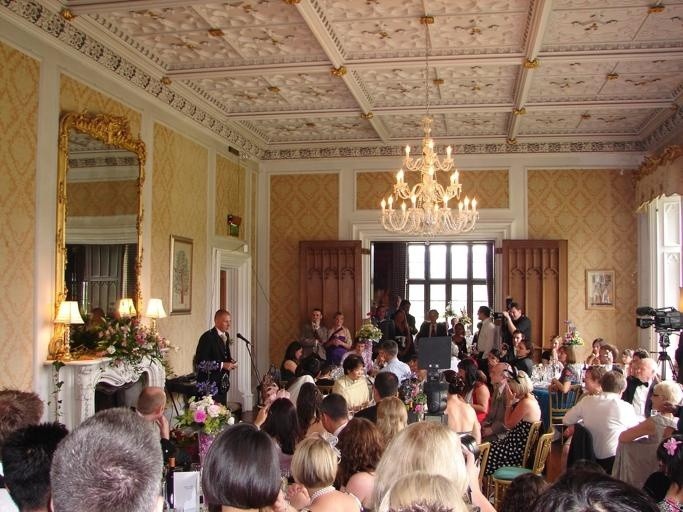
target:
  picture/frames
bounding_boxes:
[169,235,192,315]
[585,269,616,310]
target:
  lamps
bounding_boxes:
[145,297,167,333]
[113,297,137,321]
[54,300,80,359]
[378,18,478,235]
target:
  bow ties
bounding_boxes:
[637,380,648,387]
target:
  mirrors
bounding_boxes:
[50,111,146,358]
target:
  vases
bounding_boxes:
[407,409,425,423]
[199,432,213,466]
[565,343,576,364]
[363,339,374,373]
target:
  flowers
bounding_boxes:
[563,320,583,346]
[357,311,383,341]
[442,301,456,318]
[94,317,178,377]
[173,395,236,436]
[457,305,471,326]
[401,381,426,409]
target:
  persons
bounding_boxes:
[0,422,70,512]
[202,421,282,512]
[0,390,43,442]
[195,308,237,407]
[49,407,164,512]
[256,296,544,512]
[526,335,682,511]
[136,386,176,461]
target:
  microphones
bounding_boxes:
[236,332,255,347]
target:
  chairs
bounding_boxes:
[471,377,667,510]
[317,385,333,395]
[277,380,288,390]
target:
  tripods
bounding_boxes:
[657,360,676,380]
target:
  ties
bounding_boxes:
[431,325,435,337]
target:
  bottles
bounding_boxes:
[409,401,413,414]
[551,359,556,377]
[166,454,180,509]
[582,361,587,382]
